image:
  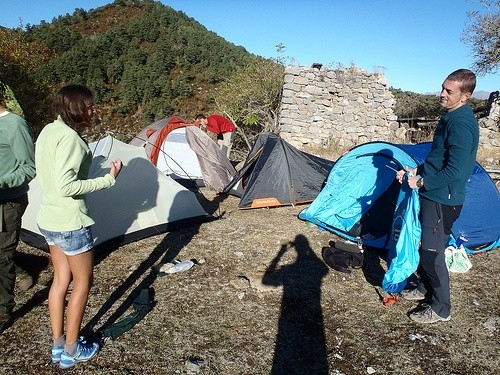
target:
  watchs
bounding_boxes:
[416,178,422,187]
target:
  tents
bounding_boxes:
[20,135,209,245]
[221,133,335,210]
[298,141,500,254]
[128,116,238,191]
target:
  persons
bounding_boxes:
[196,114,235,160]
[396,69,478,323]
[0,83,36,334]
[36,85,123,369]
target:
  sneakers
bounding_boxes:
[52,335,87,363]
[401,288,432,300]
[59,343,99,369]
[410,307,452,324]
[0,317,14,334]
